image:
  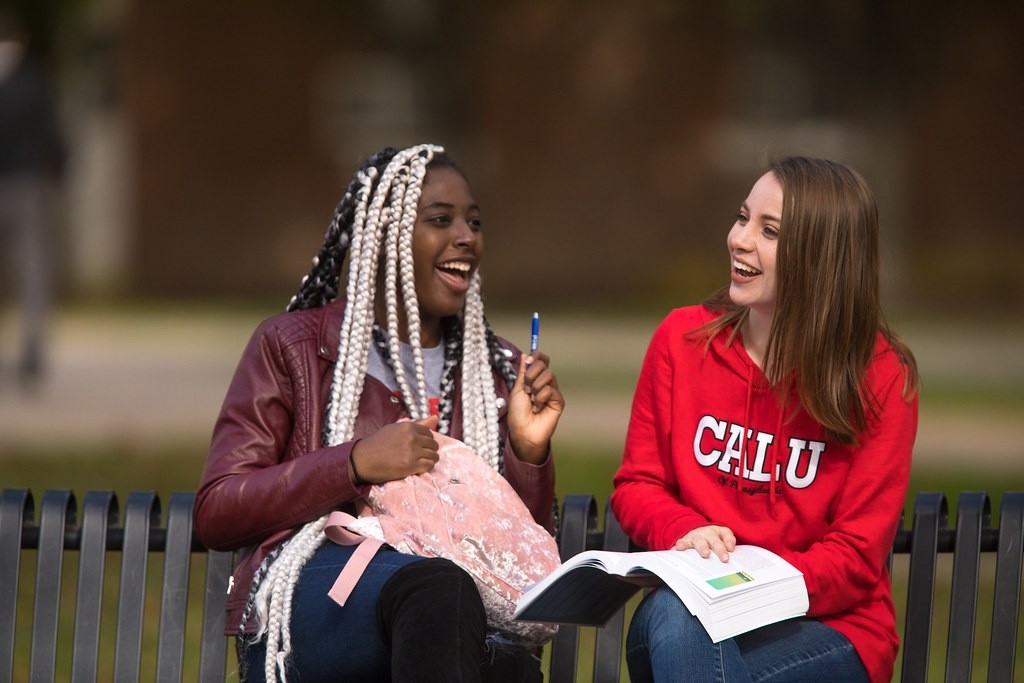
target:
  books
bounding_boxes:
[511,544,810,644]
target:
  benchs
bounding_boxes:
[0,489,1024,683]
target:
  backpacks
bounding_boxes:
[325,417,562,647]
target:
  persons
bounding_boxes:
[191,142,566,683]
[609,154,919,683]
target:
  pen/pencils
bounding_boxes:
[531,311,539,352]
